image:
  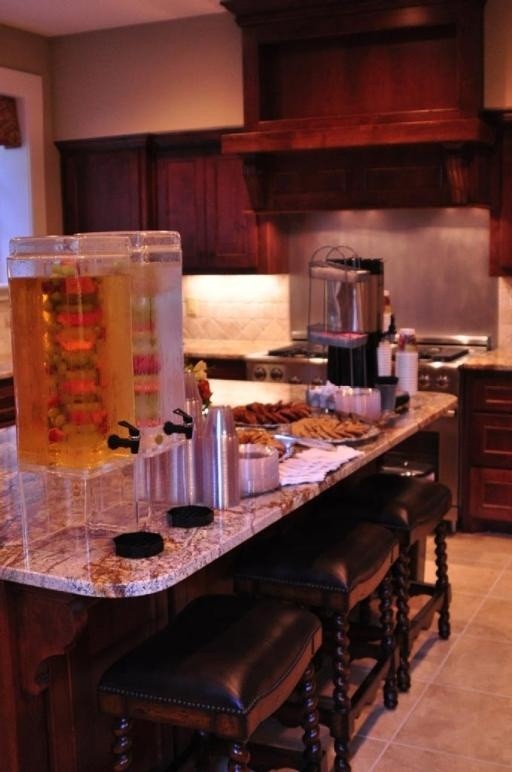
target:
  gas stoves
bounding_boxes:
[268,342,469,363]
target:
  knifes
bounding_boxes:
[272,434,335,452]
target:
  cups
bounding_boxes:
[170,370,241,510]
[373,375,399,411]
[395,327,420,357]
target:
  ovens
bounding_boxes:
[245,360,463,534]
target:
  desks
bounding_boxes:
[1,374,459,772]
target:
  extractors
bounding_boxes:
[220,2,497,214]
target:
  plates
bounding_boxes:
[276,424,381,445]
[239,443,280,497]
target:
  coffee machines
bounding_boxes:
[325,256,410,408]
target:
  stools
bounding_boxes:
[94,591,328,772]
[230,521,401,772]
[322,474,452,694]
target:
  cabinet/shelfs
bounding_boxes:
[462,375,511,533]
[151,150,256,274]
[80,149,149,231]
